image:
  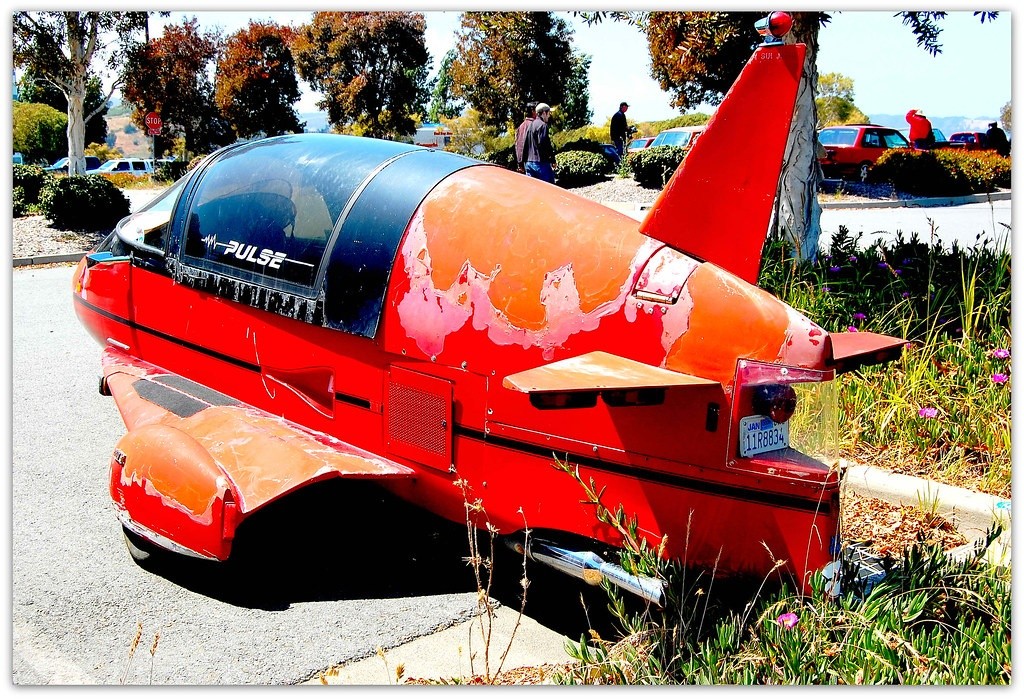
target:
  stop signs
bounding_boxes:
[146,113,162,129]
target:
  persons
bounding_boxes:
[610,102,631,157]
[985,122,1011,157]
[906,107,936,150]
[514,100,557,185]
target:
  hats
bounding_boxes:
[536,103,555,114]
[526,101,539,113]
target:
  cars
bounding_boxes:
[42,156,101,172]
[626,126,706,153]
[818,123,910,184]
[886,128,987,150]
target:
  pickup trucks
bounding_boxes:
[85,158,174,177]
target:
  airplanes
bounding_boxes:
[70,12,908,610]
